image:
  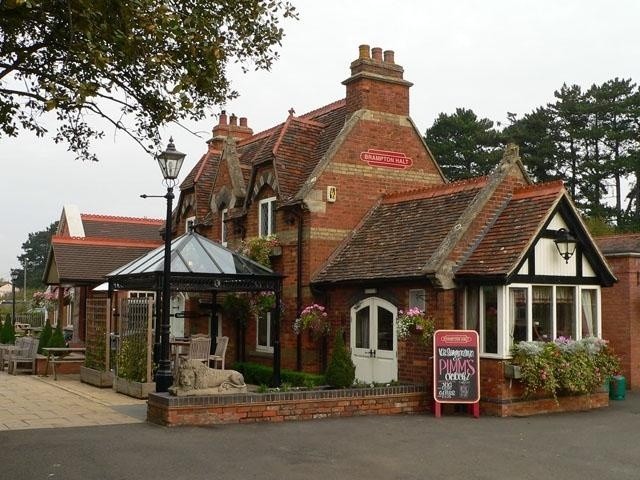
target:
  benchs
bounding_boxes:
[48,360,86,381]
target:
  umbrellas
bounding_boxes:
[92,280,124,335]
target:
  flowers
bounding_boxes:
[510,334,626,408]
[32,291,53,305]
[394,306,436,347]
[249,290,276,316]
[292,302,330,339]
[233,233,279,267]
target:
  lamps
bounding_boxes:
[553,227,580,265]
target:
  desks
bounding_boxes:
[42,347,86,381]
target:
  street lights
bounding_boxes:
[9,270,19,347]
[139,134,190,392]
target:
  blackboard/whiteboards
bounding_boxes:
[433,329,480,403]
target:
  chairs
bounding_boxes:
[0,336,40,376]
[170,333,229,370]
